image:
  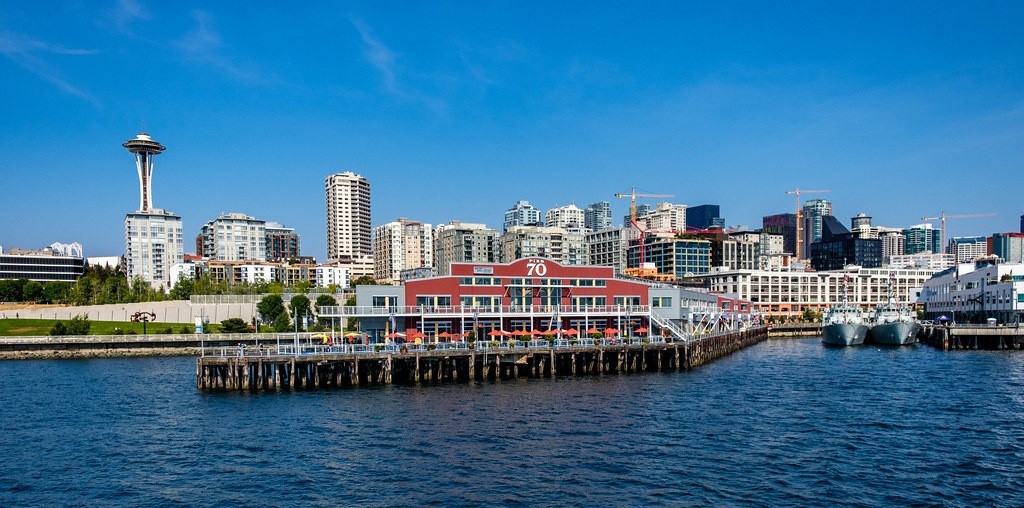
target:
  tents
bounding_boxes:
[936,315,952,325]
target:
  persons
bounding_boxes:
[237,343,248,356]
[261,343,263,356]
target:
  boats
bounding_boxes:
[820,257,868,346]
[916,254,1024,354]
[868,266,923,346]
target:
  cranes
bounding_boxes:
[613,184,678,228]
[786,185,833,261]
[919,210,1000,254]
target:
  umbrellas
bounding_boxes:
[312,327,620,349]
[634,327,648,338]
